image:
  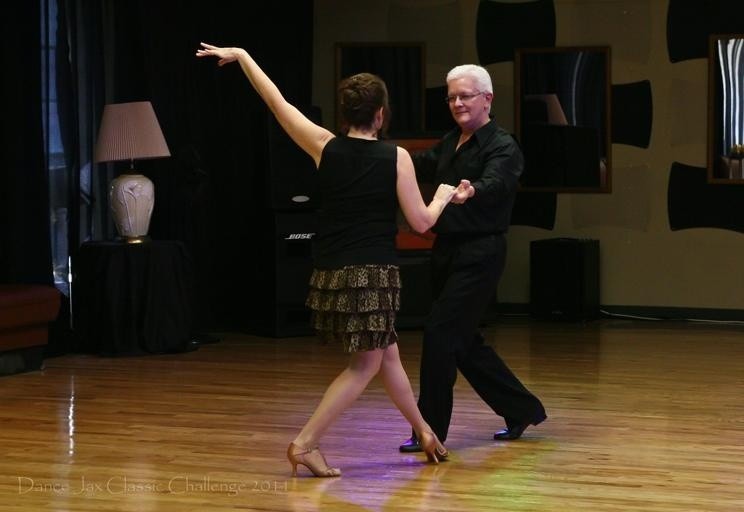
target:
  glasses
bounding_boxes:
[445,93,480,103]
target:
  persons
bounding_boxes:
[196,40,450,482]
[397,61,549,453]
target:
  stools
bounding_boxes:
[1,275,62,369]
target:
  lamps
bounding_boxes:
[95,100,173,243]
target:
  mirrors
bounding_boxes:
[513,44,613,194]
[705,30,743,186]
[336,41,429,136]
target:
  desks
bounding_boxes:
[77,241,189,357]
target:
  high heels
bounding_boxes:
[419,432,449,465]
[287,443,342,477]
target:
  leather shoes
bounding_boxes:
[400,436,443,452]
[494,414,547,440]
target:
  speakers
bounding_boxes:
[266,103,323,211]
[528,237,600,322]
